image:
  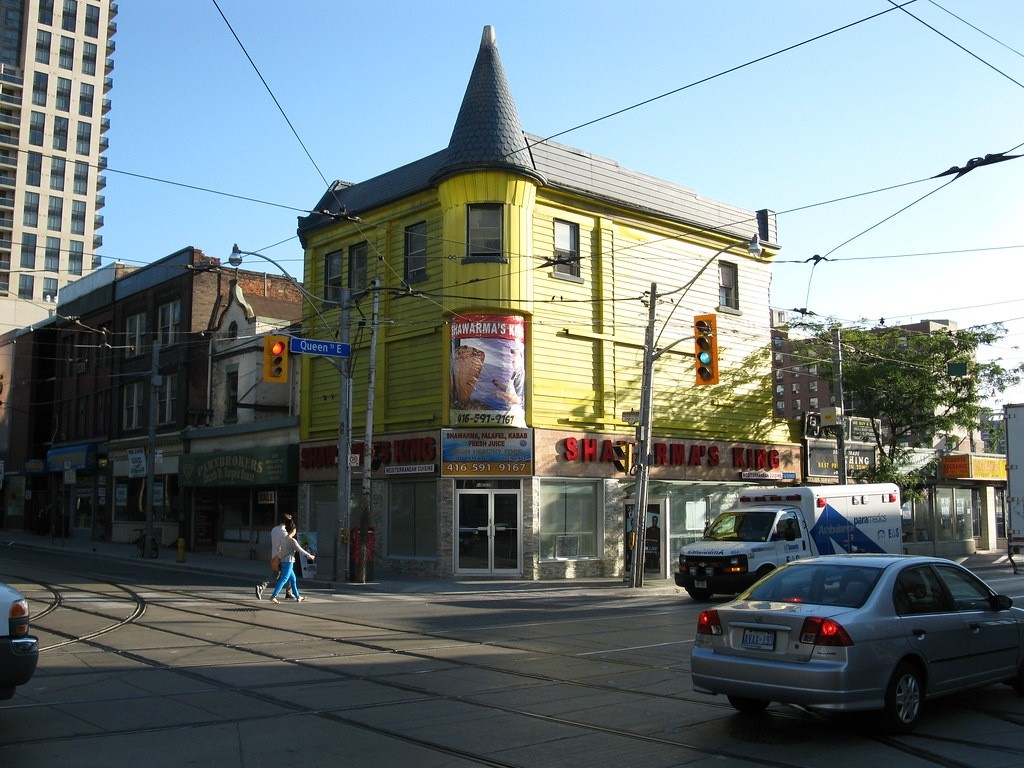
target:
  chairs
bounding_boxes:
[845,582,869,600]
[839,571,873,594]
[902,573,937,613]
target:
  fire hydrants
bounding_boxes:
[178,537,186,563]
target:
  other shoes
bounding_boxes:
[297,597,303,603]
[256,585,262,600]
[285,593,294,598]
[270,598,279,604]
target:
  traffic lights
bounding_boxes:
[262,334,288,384]
[693,314,718,384]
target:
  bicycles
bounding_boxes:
[132,526,159,559]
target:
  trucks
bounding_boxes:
[674,482,904,601]
[1002,403,1024,549]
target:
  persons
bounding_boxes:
[646,516,660,573]
[255,514,315,604]
[626,518,634,572]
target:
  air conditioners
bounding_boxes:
[77,363,86,374]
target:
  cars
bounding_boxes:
[692,553,1024,736]
[0,580,39,702]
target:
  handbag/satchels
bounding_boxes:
[271,556,281,572]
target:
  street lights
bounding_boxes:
[229,243,351,581]
[629,233,760,588]
